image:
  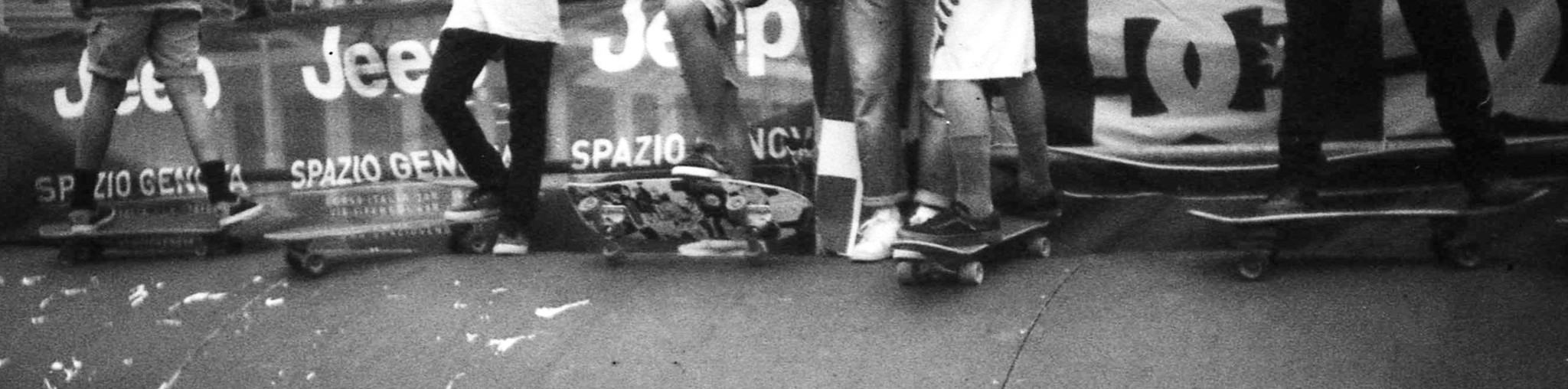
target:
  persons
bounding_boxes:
[1256,0,1542,216]
[663,0,1062,260]
[68,0,263,231]
[423,0,561,255]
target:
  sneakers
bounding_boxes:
[997,186,1062,218]
[899,201,1002,241]
[851,209,905,262]
[492,220,530,255]
[909,205,938,226]
[443,184,505,222]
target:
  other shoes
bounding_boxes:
[68,205,114,232]
[678,239,769,257]
[1465,173,1550,207]
[213,195,264,230]
[671,152,735,180]
[1257,180,1324,219]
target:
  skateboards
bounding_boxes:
[560,175,817,263]
[888,220,1053,288]
[261,212,500,277]
[37,211,300,269]
[1184,184,1552,280]
